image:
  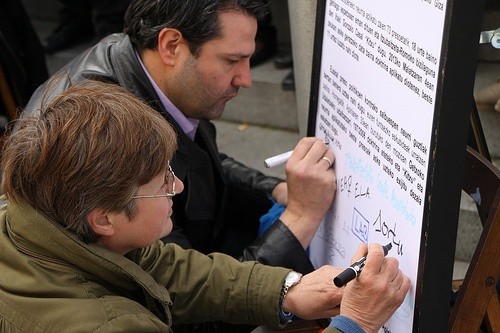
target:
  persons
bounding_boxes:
[42,0,297,91]
[5,82,416,333]
[4,2,337,333]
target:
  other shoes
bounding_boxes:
[282,72,294,91]
[274,49,292,68]
[46,26,98,54]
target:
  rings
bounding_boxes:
[323,156,333,167]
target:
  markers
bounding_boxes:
[332,243,393,288]
[263,140,328,169]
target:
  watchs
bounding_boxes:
[279,270,303,317]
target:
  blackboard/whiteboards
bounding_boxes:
[293,1,487,333]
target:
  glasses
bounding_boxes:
[132,160,177,200]
